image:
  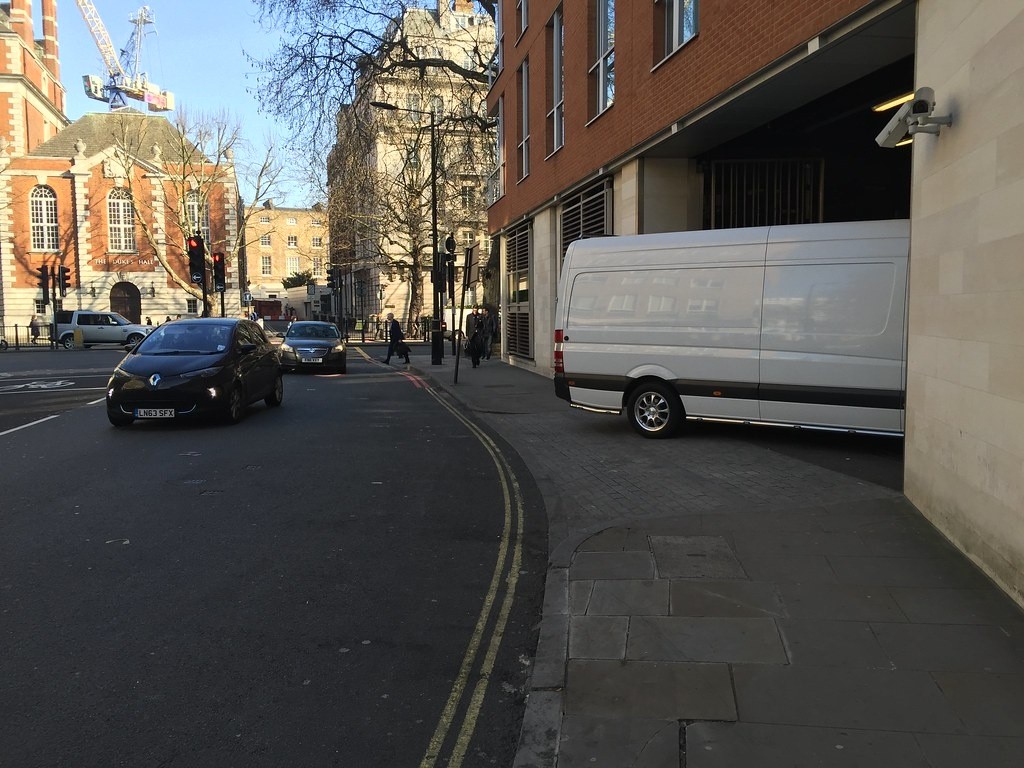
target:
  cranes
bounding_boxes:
[74,1,177,111]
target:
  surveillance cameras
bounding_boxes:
[875,86,936,148]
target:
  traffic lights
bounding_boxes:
[213,251,224,281]
[36,265,48,287]
[186,237,204,270]
[60,265,71,288]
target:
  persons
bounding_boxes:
[177,314,181,319]
[95,316,109,325]
[146,317,152,325]
[288,315,296,329]
[381,313,410,365]
[29,315,40,344]
[299,326,335,338]
[165,316,171,322]
[467,305,497,368]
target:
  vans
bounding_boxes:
[551,217,911,441]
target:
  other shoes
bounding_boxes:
[403,361,410,364]
[381,360,389,364]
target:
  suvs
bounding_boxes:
[48,310,155,349]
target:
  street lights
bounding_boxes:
[369,100,443,365]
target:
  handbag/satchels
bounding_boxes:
[464,339,472,354]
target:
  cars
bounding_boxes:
[104,317,284,428]
[276,320,348,376]
